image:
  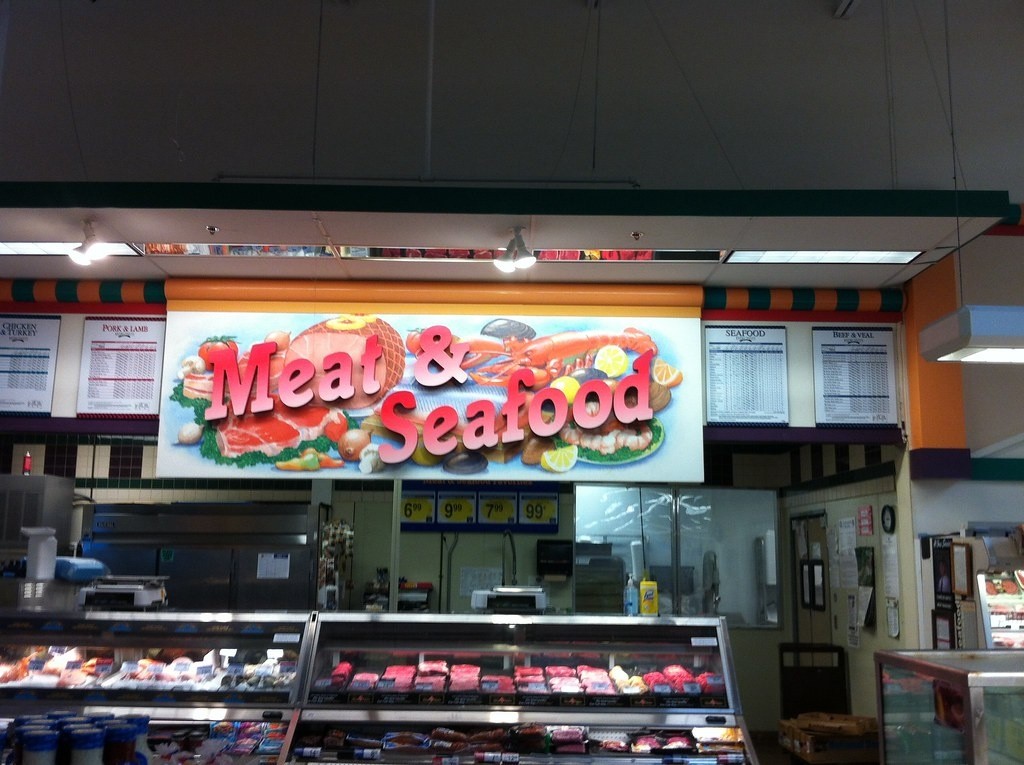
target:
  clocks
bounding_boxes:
[881,504,896,534]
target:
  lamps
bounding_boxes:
[915,0,1023,366]
[69,219,110,266]
[492,226,537,274]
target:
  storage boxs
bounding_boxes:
[777,710,878,764]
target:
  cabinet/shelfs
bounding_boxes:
[1,610,761,765]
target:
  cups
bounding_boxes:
[1,710,154,765]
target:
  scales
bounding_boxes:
[472,586,548,615]
[80,574,171,612]
